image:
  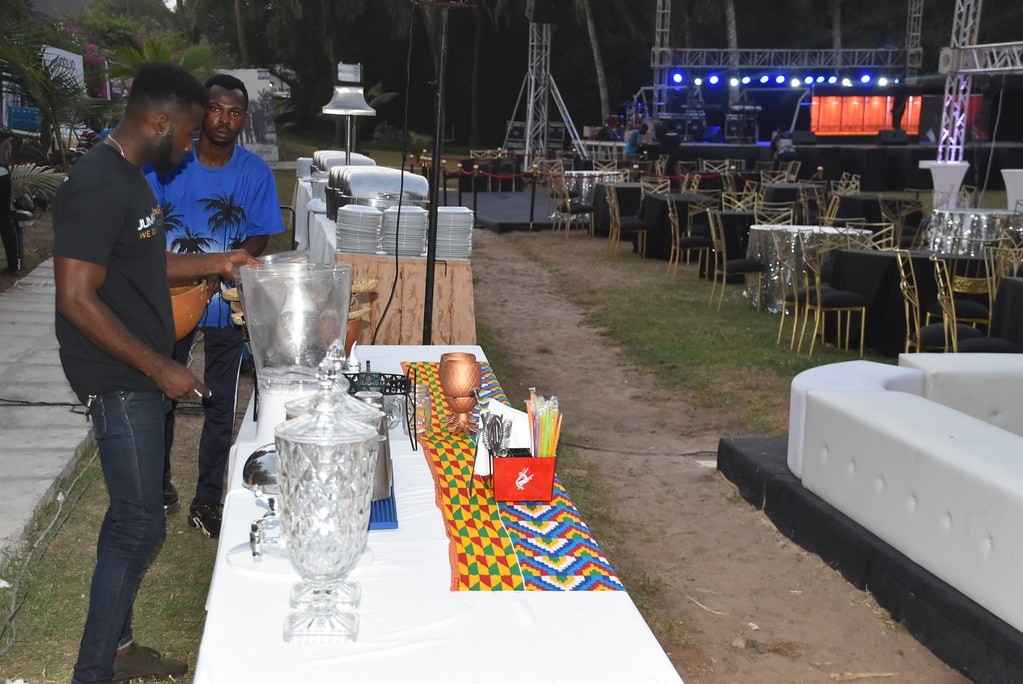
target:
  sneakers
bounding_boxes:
[113,641,188,680]
[163,482,180,517]
[188,497,224,537]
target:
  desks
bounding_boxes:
[289,178,477,346]
[547,170,625,222]
[631,191,719,262]
[699,211,821,285]
[742,225,873,316]
[587,181,652,241]
[927,208,1023,257]
[823,248,998,359]
[989,276,1023,350]
[694,168,762,210]
[193,345,684,684]
[833,194,923,249]
[761,182,831,219]
[458,157,522,193]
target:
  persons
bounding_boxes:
[54,67,261,684]
[772,124,793,160]
[100,119,114,138]
[141,74,283,539]
[624,123,648,155]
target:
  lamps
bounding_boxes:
[336,61,365,152]
[321,85,376,166]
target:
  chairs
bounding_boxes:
[470,150,1023,361]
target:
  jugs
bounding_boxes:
[353,390,391,500]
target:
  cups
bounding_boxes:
[384,394,402,428]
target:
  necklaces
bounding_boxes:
[108,134,125,158]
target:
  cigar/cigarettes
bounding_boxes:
[193,388,202,397]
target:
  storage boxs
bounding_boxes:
[724,114,759,144]
[492,454,557,502]
[657,111,707,139]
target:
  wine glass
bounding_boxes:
[275,376,383,640]
[439,352,482,435]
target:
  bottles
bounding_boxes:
[403,384,431,435]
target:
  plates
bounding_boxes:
[383,206,428,257]
[336,204,383,255]
[436,206,474,258]
